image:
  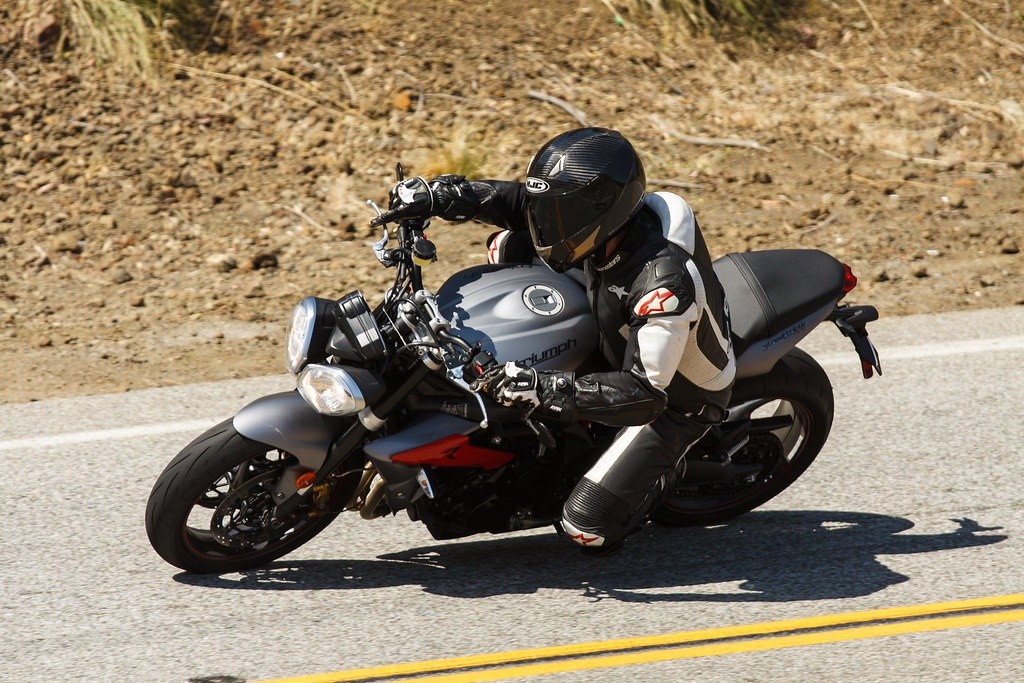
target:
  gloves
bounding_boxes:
[470,360,578,428]
[367,173,476,229]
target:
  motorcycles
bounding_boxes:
[144,160,882,574]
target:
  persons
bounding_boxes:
[371,128,738,549]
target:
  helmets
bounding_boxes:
[522,126,647,274]
